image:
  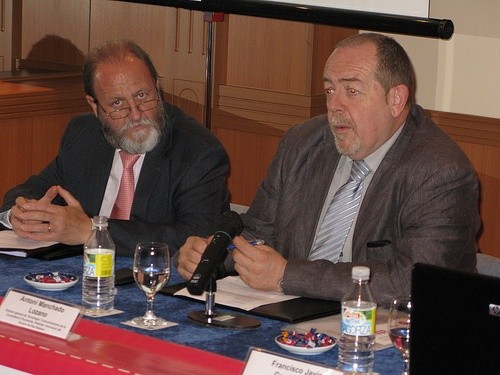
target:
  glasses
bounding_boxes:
[95,78,159,121]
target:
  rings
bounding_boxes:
[48,223,51,233]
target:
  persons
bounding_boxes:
[177,33,482,307]
[0,40,231,257]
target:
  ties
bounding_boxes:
[307,158,372,264]
[108,151,140,220]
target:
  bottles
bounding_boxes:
[82,215,115,315]
[337,266,377,375]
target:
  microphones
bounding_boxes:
[187,210,245,297]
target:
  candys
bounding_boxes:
[28,272,71,283]
[280,327,335,348]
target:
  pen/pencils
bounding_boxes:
[225,238,266,251]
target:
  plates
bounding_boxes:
[275,332,340,356]
[24,272,79,291]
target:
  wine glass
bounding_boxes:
[388,294,411,375]
[130,241,171,329]
